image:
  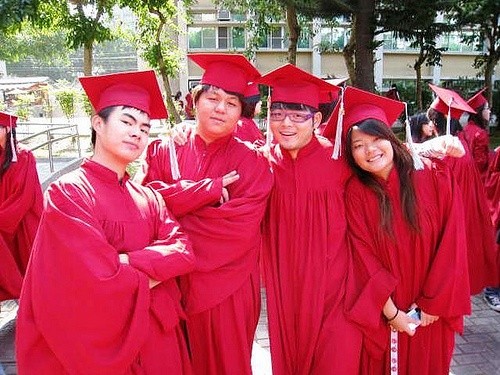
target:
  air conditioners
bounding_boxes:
[217,10,231,21]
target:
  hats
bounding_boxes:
[253,63,344,160]
[79,70,182,180]
[467,86,489,110]
[321,86,425,171]
[0,112,18,162]
[428,84,477,135]
[188,55,278,173]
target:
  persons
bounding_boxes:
[141,54,274,375]
[320,85,470,375]
[0,110,44,303]
[174,84,263,120]
[169,64,465,375]
[14,69,199,375]
[401,83,500,313]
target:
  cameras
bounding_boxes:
[406,307,422,330]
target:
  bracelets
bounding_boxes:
[387,308,399,321]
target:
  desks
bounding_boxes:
[0,76,49,102]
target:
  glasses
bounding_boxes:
[270,112,314,121]
[484,106,489,110]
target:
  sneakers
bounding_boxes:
[481,288,500,311]
